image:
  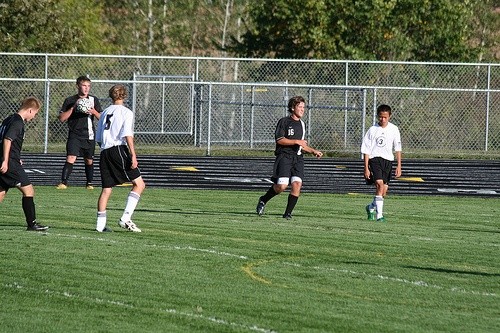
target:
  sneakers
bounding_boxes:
[95,227,113,232]
[118,218,142,232]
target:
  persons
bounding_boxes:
[57,76,102,189]
[360,104,402,223]
[256,96,323,219]
[95,85,145,233]
[0,97,48,232]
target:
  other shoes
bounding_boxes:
[283,213,292,220]
[256,196,266,215]
[56,183,68,190]
[86,186,94,190]
[365,205,376,221]
[26,221,49,231]
[377,216,384,222]
